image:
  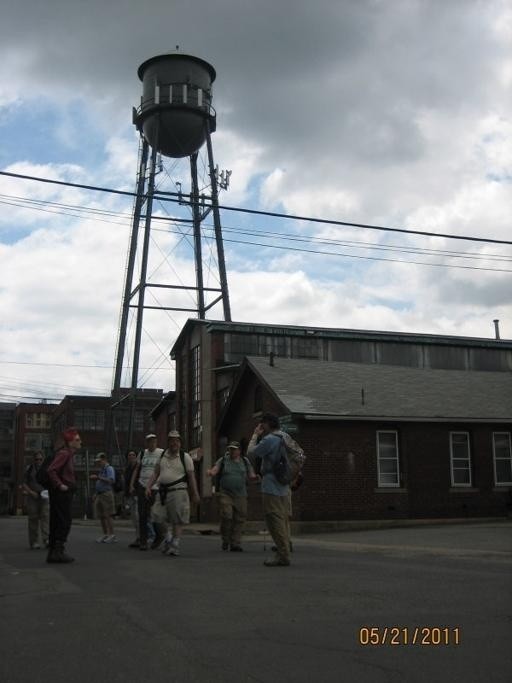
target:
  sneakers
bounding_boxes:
[150,533,166,550]
[30,537,50,550]
[46,549,75,564]
[263,558,290,567]
[271,544,293,552]
[139,535,149,550]
[159,541,181,556]
[221,541,246,552]
[95,534,119,544]
[128,538,141,548]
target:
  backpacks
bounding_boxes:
[37,445,72,488]
[157,446,201,496]
[211,455,250,493]
[267,428,308,491]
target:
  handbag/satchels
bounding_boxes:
[127,462,145,489]
[47,483,78,507]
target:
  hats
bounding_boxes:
[254,411,280,423]
[225,439,241,449]
[143,431,159,440]
[94,451,107,462]
[167,429,183,439]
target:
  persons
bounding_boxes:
[271,477,293,553]
[207,442,261,552]
[21,449,50,550]
[46,429,82,563]
[89,452,118,543]
[130,433,165,550]
[144,431,201,556]
[247,414,296,567]
[123,450,155,547]
